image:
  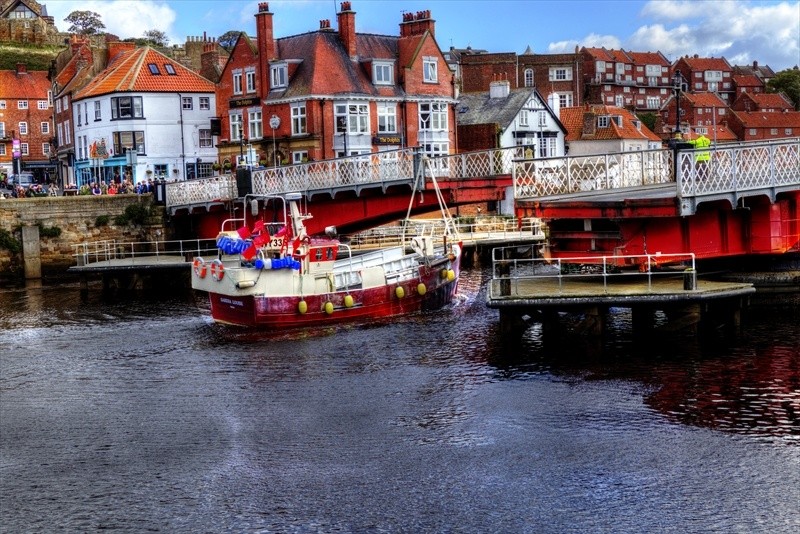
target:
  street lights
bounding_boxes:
[668,68,686,149]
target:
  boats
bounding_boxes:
[189,152,464,330]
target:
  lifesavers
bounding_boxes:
[193,257,206,278]
[210,258,225,281]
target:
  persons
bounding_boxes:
[79,177,153,196]
[686,131,712,182]
[15,183,59,197]
[63,183,78,196]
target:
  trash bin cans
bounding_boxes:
[683,268,697,290]
[500,274,511,295]
[533,222,539,235]
[339,235,351,250]
[185,250,193,261]
[668,143,696,182]
